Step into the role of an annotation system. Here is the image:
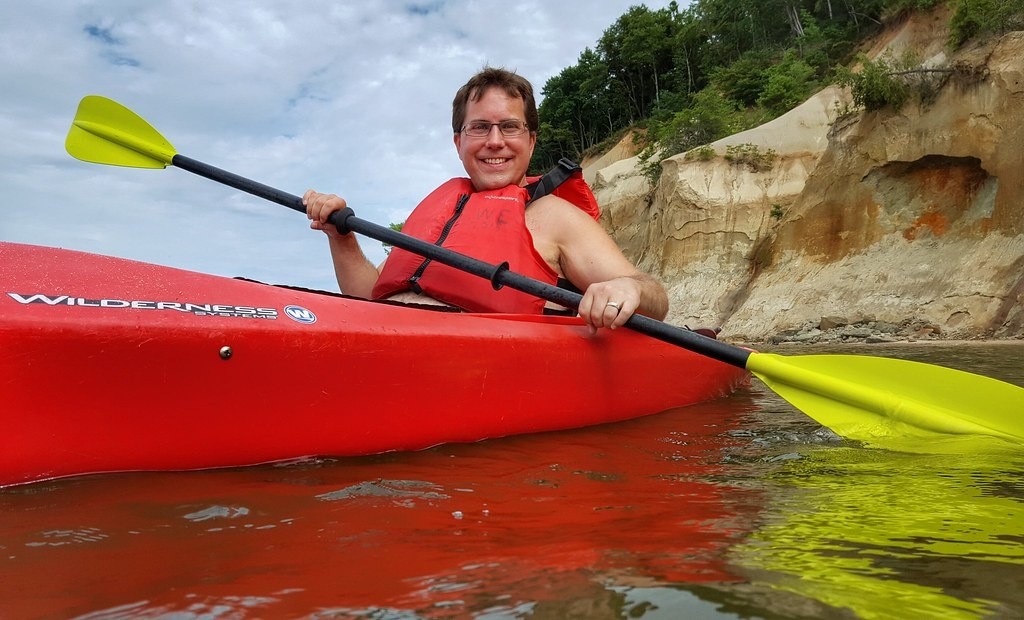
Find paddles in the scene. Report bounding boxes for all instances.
[63,95,1023,441]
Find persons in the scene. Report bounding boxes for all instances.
[302,58,669,335]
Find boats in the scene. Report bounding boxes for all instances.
[0,241,759,489]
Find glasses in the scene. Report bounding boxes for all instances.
[458,119,530,137]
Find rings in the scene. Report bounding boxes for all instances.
[606,301,620,310]
